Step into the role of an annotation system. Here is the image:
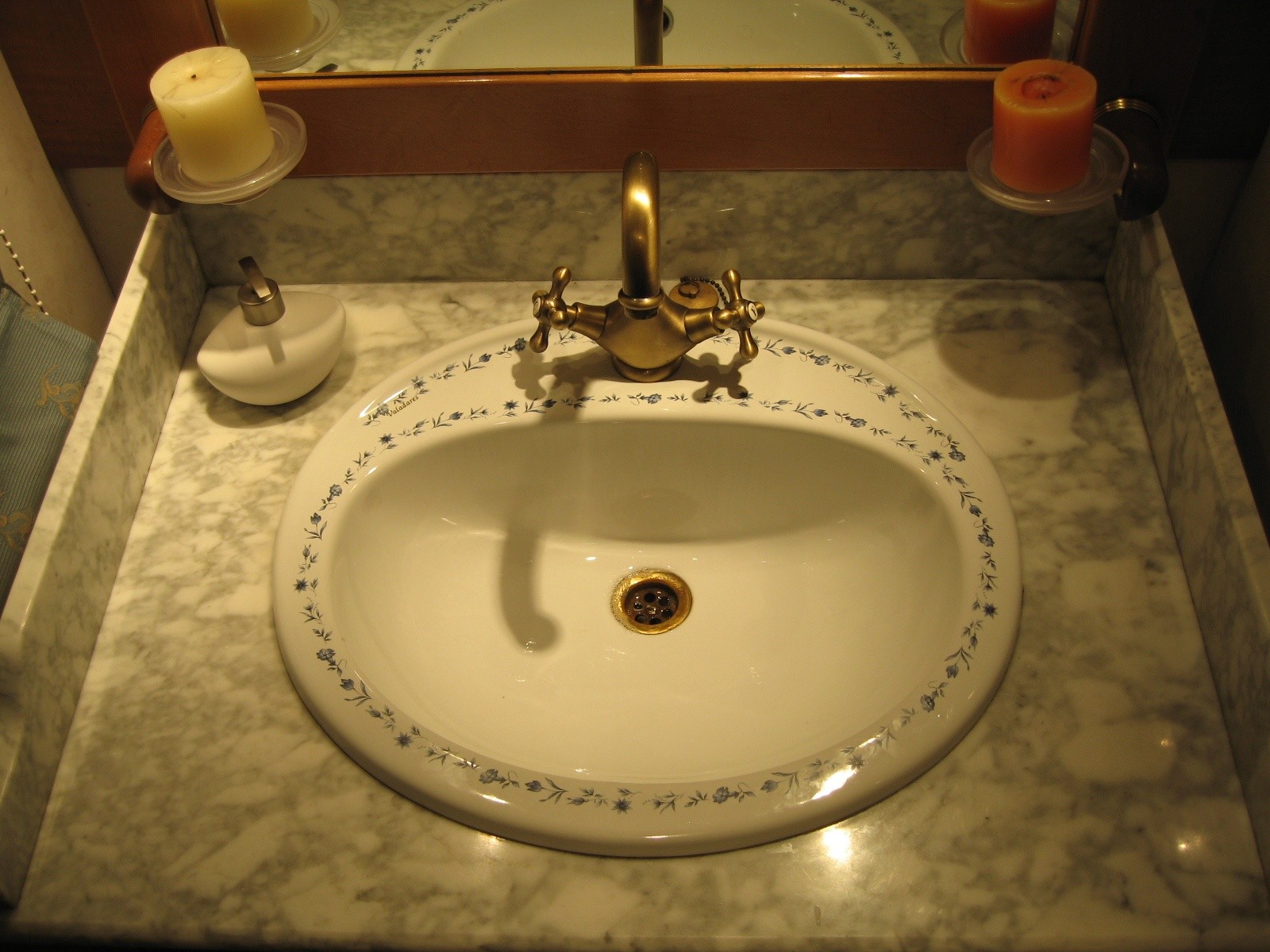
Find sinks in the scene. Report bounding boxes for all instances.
[393,0,924,71]
[267,400,1028,860]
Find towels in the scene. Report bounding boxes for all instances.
[0,283,100,616]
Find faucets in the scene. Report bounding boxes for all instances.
[528,148,766,385]
[633,0,663,66]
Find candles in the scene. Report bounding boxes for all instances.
[963,0,1054,65]
[215,1,313,59]
[151,47,275,183]
[991,58,1097,194]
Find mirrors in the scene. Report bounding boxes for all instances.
[81,0,1214,177]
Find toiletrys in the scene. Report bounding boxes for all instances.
[196,255,346,406]
